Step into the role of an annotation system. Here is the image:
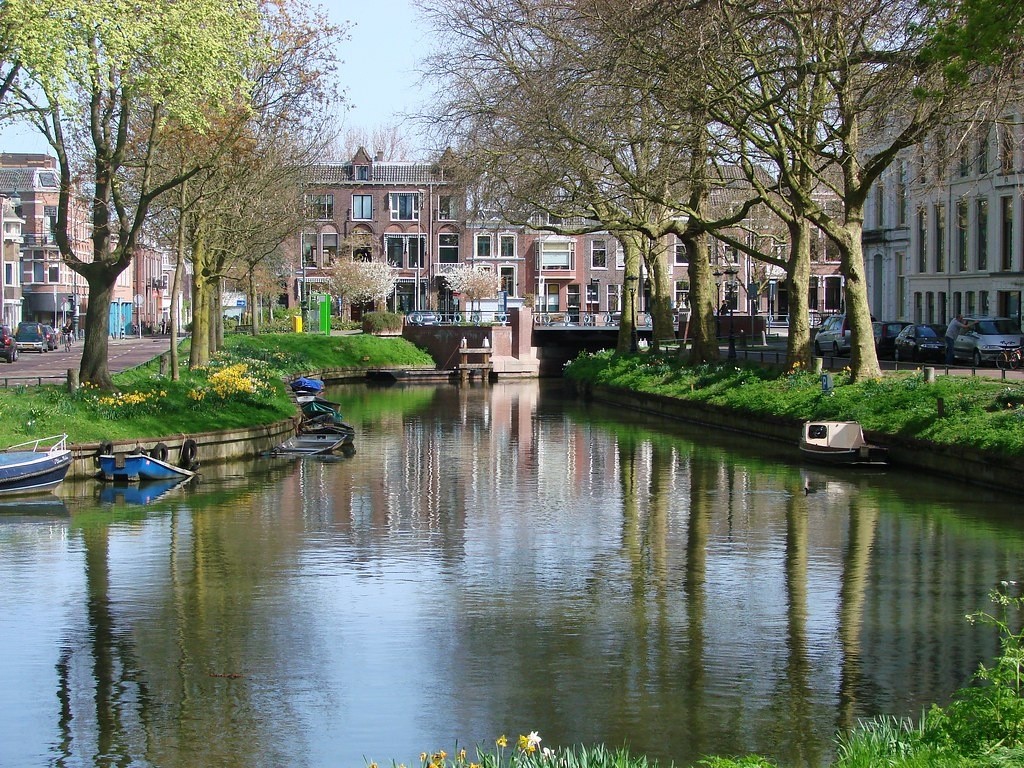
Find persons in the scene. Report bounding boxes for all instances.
[718,300,729,317]
[945,313,979,364]
[62,318,72,345]
[161,319,166,335]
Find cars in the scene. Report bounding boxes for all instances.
[872,321,914,360]
[894,324,948,363]
[44,325,58,351]
[407,311,437,325]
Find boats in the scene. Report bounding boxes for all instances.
[290,377,325,392]
[259,433,347,458]
[799,420,890,471]
[99,452,195,482]
[302,400,341,419]
[0,434,72,497]
[295,391,327,405]
[300,412,355,445]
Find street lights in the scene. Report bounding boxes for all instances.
[712,269,724,341]
[0,187,21,327]
[626,274,639,352]
[723,268,739,359]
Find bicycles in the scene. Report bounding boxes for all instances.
[996,340,1022,370]
[63,333,71,353]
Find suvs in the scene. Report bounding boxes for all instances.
[0,324,19,363]
[15,322,48,353]
[946,313,1024,367]
[814,314,878,358]
